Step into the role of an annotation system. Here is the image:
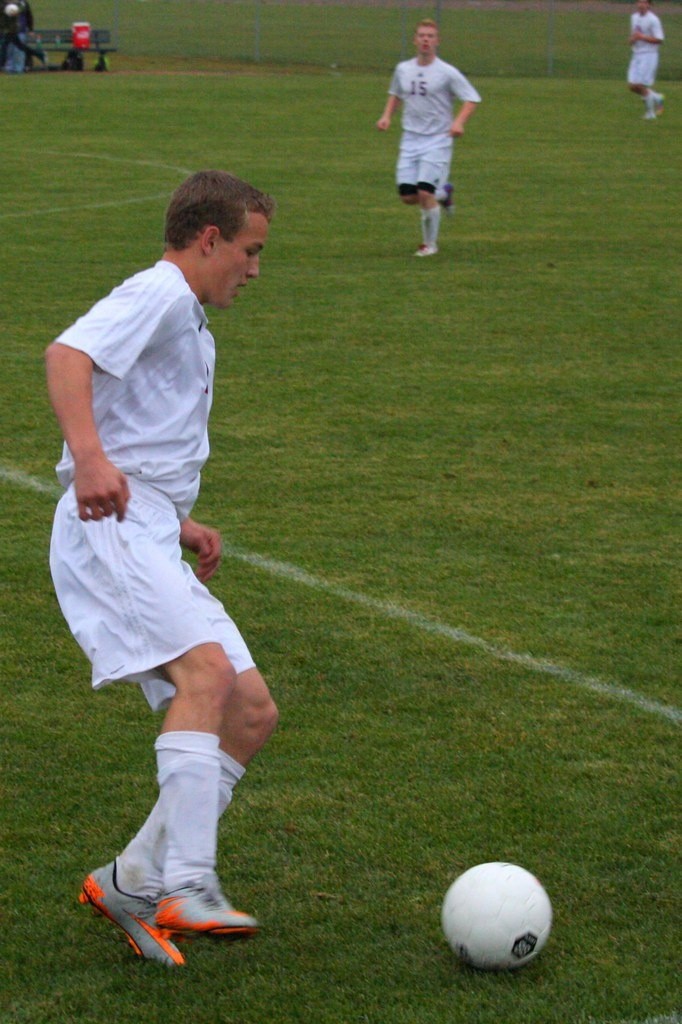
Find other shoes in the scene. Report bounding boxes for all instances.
[415,245,437,258]
[657,95,666,115]
[644,114,655,120]
[440,186,455,217]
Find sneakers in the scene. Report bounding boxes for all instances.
[155,873,261,938]
[79,856,185,969]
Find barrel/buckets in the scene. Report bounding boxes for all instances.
[71,21,92,48]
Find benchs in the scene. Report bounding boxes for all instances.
[27,30,118,72]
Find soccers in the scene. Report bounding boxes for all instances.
[439,860,555,971]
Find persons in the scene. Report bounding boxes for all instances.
[625,0,665,121]
[46,168,280,966]
[0,0,47,72]
[375,18,482,258]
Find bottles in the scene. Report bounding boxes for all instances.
[55,35,60,47]
[36,35,42,48]
